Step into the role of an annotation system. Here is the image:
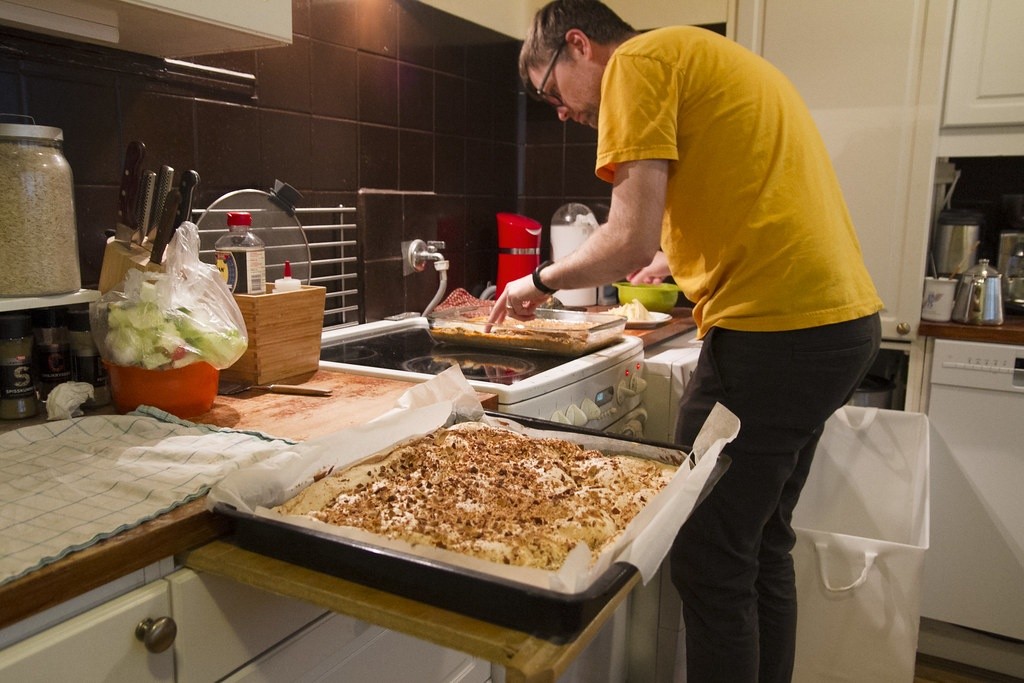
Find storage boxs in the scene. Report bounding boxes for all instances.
[224,281,327,387]
[787,405,930,681]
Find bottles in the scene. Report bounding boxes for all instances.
[272,261,306,296]
[213,212,267,298]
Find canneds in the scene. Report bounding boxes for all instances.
[0,123,81,296]
[0,307,113,422]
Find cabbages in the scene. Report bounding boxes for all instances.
[101,280,250,369]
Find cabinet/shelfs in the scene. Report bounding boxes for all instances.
[734,0,954,344]
[0,0,292,59]
[624,327,703,682]
[0,579,175,683]
[161,566,492,683]
[935,0,1023,157]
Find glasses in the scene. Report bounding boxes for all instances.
[536,38,565,107]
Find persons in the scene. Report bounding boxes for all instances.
[486,0,885,683]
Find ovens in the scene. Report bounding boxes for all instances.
[494,337,647,683]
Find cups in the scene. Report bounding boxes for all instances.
[920,276,960,321]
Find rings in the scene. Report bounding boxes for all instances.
[505,303,513,309]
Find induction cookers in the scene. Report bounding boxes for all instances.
[314,311,644,391]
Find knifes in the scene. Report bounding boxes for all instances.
[116,139,147,250]
[169,169,203,241]
[130,170,156,246]
[149,189,180,264]
[151,163,176,238]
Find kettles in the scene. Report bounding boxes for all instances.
[492,210,544,306]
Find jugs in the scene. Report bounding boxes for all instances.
[952,258,1005,326]
[933,211,982,277]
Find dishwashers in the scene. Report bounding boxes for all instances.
[915,328,1024,683]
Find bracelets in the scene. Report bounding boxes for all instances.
[531,258,560,294]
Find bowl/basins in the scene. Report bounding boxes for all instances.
[99,359,221,418]
[848,376,896,411]
[611,281,685,313]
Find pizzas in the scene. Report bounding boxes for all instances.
[279,422,682,575]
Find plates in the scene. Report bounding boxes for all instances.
[595,310,673,329]
